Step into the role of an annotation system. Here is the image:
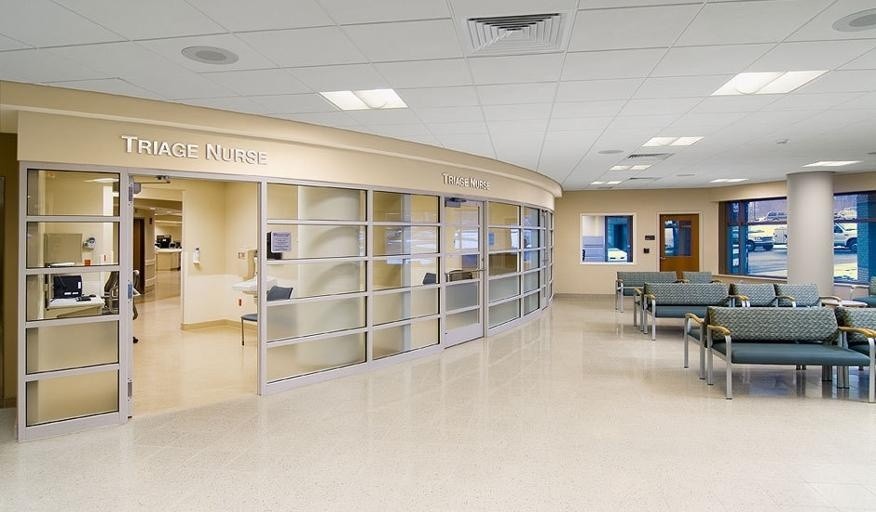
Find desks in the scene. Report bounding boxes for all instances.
[43,294,103,320]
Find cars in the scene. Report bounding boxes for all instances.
[607,249,628,262]
[664,243,674,256]
[733,208,858,254]
[386,226,528,269]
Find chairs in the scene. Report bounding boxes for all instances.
[101,270,140,345]
[240,286,292,346]
[421,269,473,286]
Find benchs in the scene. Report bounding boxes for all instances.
[614,268,876,403]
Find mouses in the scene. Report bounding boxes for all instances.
[90,293,96,297]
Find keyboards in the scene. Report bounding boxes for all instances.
[77,295,91,301]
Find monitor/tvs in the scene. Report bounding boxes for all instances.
[54,275,82,298]
